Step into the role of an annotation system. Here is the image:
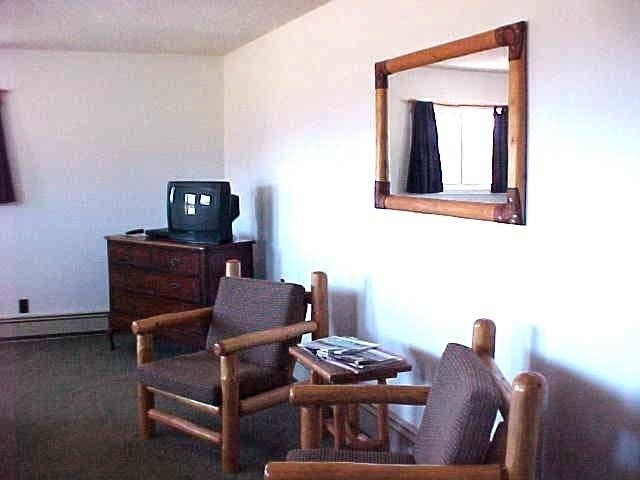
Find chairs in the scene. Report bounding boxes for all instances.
[264,318,546,479]
[131,258,328,474]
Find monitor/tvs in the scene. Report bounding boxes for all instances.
[167,181,239,243]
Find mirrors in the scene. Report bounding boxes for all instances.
[374,21,527,227]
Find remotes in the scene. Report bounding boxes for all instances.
[127,229,145,233]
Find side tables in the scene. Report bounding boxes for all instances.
[288,334,412,451]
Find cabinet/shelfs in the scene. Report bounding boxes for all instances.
[103,231,256,350]
